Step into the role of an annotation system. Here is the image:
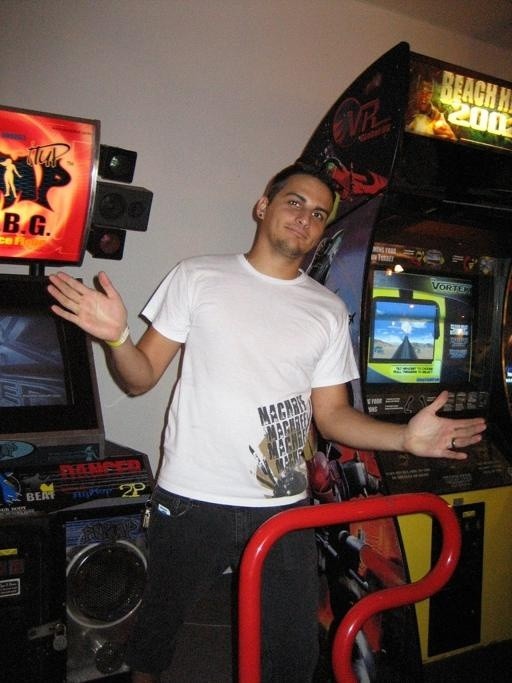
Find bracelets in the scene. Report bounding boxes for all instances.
[105,327,130,347]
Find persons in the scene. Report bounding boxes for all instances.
[47,163,488,683]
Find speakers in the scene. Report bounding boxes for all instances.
[86,227,126,261]
[98,143,138,184]
[91,181,153,231]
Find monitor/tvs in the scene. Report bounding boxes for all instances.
[365,270,486,391]
[1,298,98,434]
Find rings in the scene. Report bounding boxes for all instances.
[452,437,456,448]
